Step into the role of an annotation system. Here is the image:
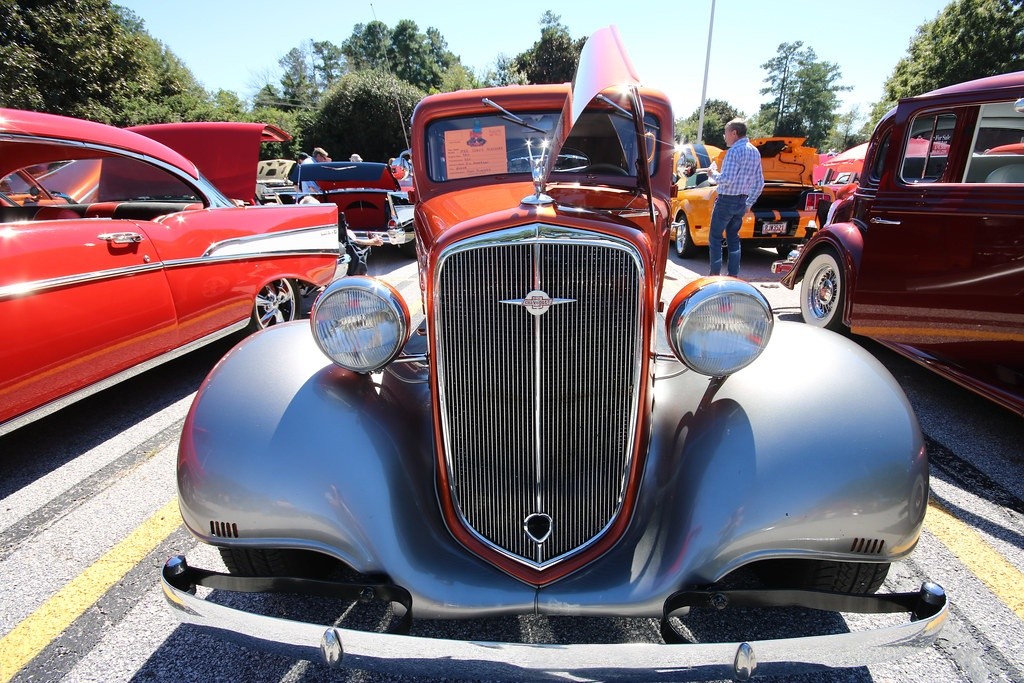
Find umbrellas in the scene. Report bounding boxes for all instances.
[821,137,951,174]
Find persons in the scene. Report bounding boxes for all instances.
[350,154,362,163]
[708,121,764,278]
[389,151,412,166]
[288,147,332,186]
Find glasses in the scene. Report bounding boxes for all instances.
[321,155,326,160]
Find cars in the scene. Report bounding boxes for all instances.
[0,104,352,437]
[665,136,834,259]
[155,30,951,670]
[287,158,420,258]
[770,66,1024,420]
